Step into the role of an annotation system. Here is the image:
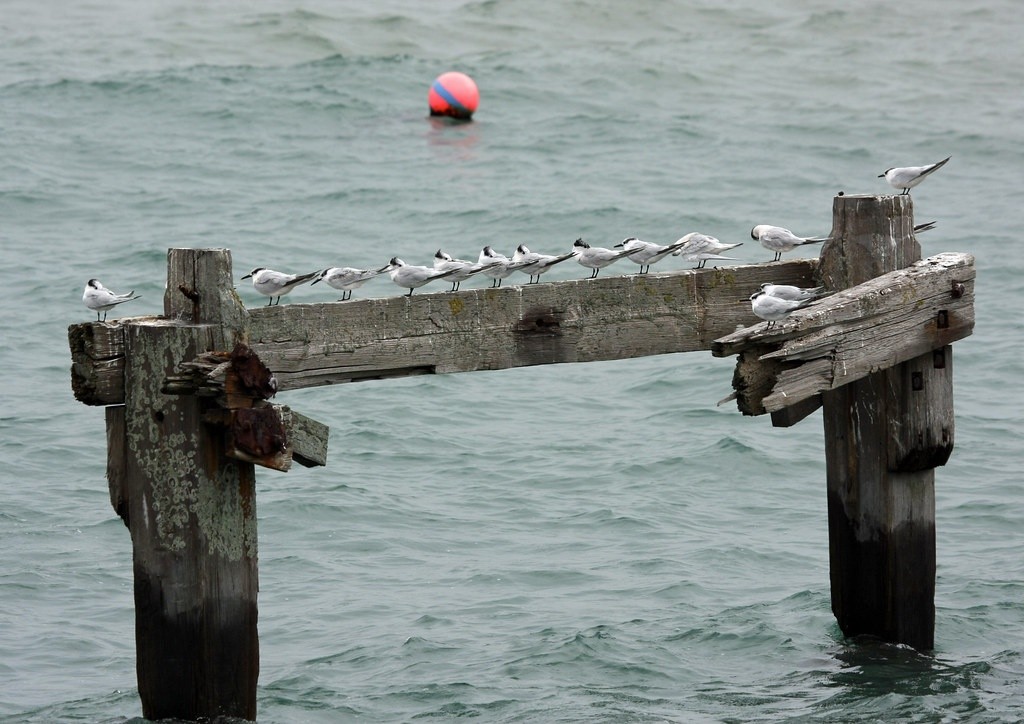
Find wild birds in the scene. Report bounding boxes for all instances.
[510,244,580,284]
[750,224,835,263]
[378,256,465,299]
[81,275,141,326]
[613,236,691,276]
[476,244,540,288]
[739,282,825,331]
[239,264,330,306]
[672,231,745,269]
[571,237,647,278]
[914,219,938,235]
[877,155,953,195]
[433,247,502,292]
[309,263,401,302]
